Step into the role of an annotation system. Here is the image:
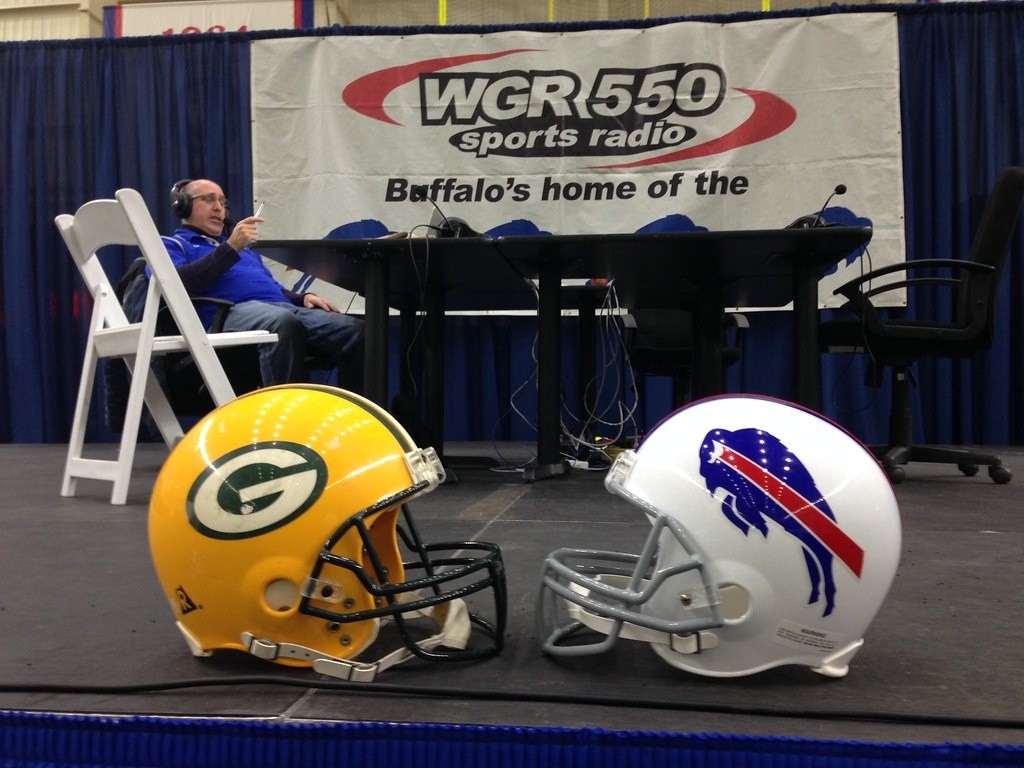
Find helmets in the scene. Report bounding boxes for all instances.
[536,393,902,677]
[148,383,508,682]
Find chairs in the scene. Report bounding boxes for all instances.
[55,188,280,506]
[129,252,334,414]
[818,166,1024,483]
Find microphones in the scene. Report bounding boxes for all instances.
[811,184,847,227]
[414,186,454,231]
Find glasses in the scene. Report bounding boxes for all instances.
[192,194,230,206]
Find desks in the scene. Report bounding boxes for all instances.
[248,225,872,482]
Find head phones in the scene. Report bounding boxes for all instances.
[438,212,490,238]
[170,179,193,221]
[784,214,825,231]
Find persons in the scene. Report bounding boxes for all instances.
[145,177,366,395]
